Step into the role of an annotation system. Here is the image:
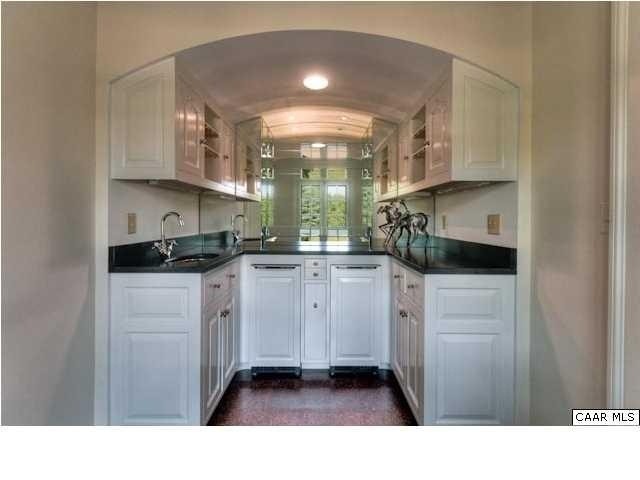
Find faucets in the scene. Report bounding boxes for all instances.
[152,211,184,260]
[230,214,249,248]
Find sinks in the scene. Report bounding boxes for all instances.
[242,237,261,242]
[164,252,220,264]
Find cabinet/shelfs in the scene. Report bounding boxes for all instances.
[329,256,387,374]
[243,254,301,375]
[301,255,328,370]
[111,258,241,425]
[109,57,261,202]
[374,56,520,201]
[389,257,515,425]
